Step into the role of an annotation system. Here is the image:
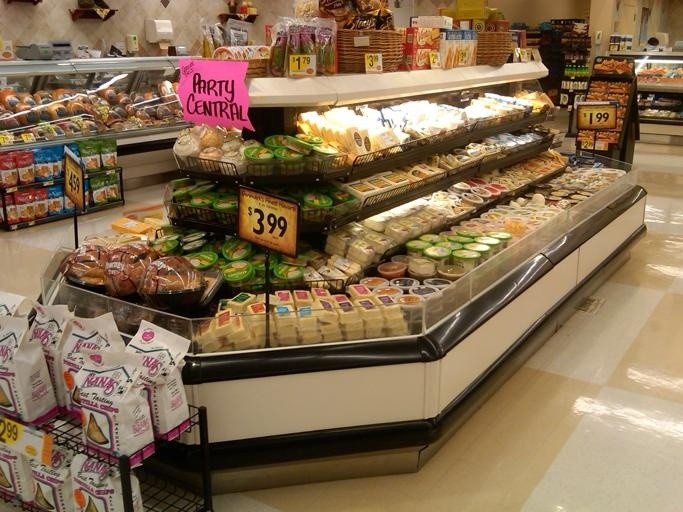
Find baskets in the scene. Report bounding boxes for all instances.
[478,30,512,68]
[337,28,405,72]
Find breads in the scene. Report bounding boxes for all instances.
[60,239,207,296]
